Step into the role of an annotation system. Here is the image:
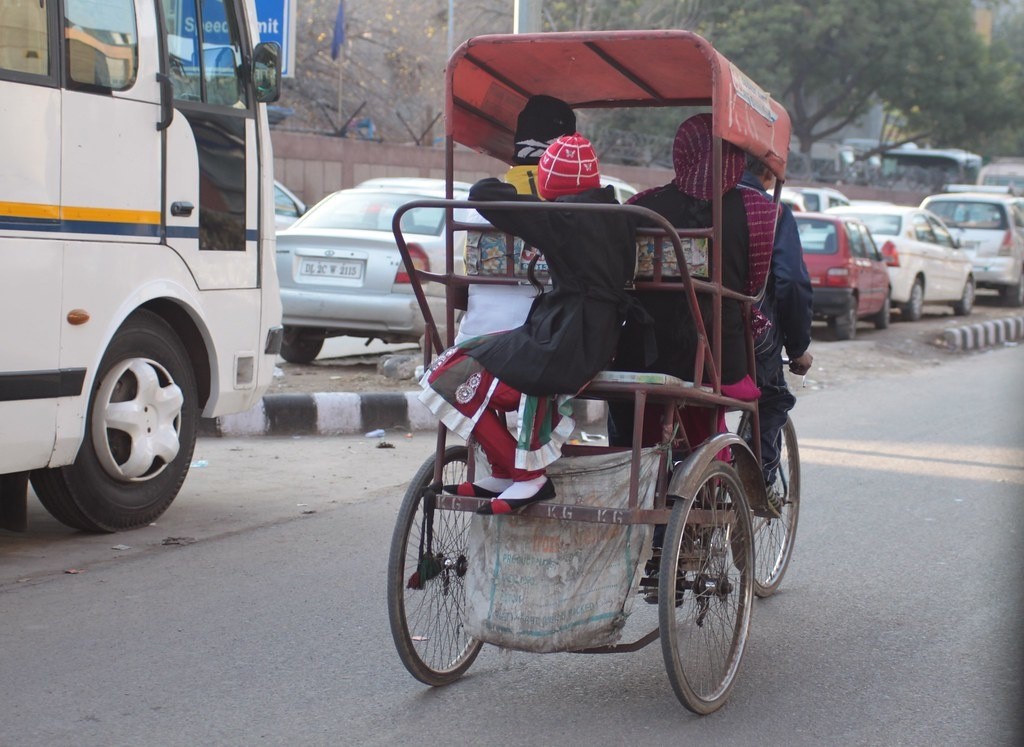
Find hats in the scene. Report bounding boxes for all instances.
[512,95,577,163]
[538,133,601,200]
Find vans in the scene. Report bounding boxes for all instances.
[919,192,1024,306]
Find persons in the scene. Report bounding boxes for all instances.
[415,94,638,516]
[608,113,817,608]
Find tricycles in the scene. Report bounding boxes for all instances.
[388,29,800,715]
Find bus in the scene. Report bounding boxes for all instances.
[786,137,982,194]
[0,0,284,534]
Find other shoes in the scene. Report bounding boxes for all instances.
[753,485,781,518]
[477,478,555,515]
[444,483,504,497]
[644,569,684,607]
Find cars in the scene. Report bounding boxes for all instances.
[274,174,638,363]
[791,211,892,339]
[766,186,852,212]
[976,162,1024,197]
[824,205,976,321]
